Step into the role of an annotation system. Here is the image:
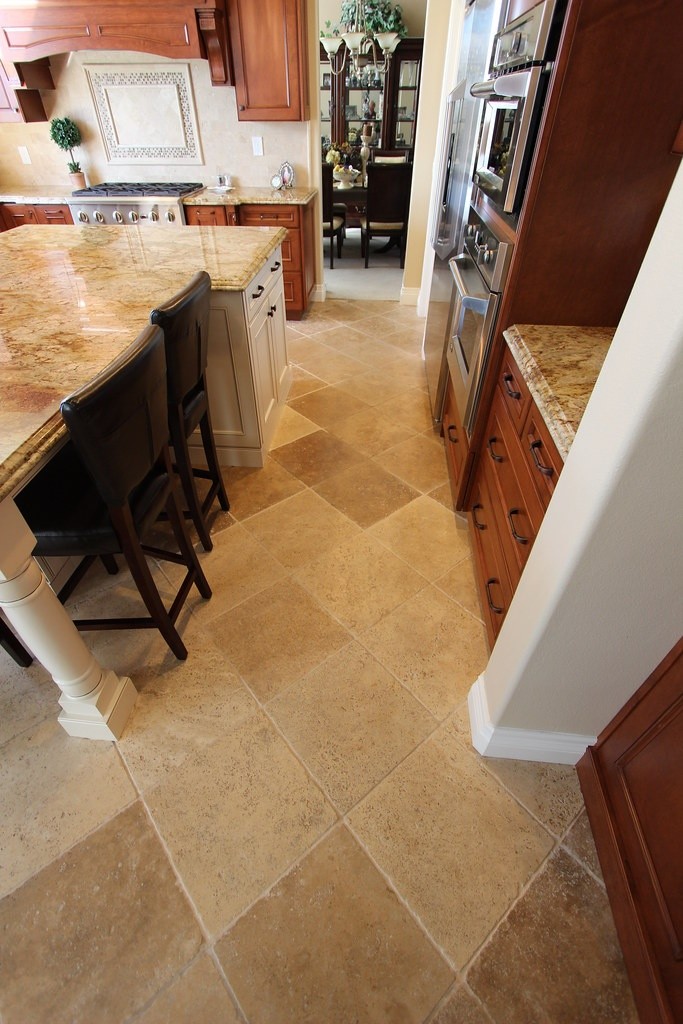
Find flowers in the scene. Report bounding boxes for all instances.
[333,161,358,173]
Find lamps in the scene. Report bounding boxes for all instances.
[319,0,402,75]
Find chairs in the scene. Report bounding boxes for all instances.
[0,319,216,669]
[358,161,413,269]
[156,265,236,552]
[323,162,346,270]
[370,148,411,163]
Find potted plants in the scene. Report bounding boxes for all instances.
[47,115,86,189]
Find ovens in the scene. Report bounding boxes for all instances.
[445,182,524,450]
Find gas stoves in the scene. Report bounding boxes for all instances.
[66,182,204,228]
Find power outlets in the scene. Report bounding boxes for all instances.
[18,146,32,165]
[251,136,265,156]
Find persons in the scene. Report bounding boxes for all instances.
[282,167,290,184]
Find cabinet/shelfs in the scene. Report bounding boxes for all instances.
[440,370,474,515]
[0,203,73,230]
[173,243,287,471]
[238,204,316,322]
[459,339,566,653]
[188,207,238,226]
[0,0,235,124]
[224,0,314,122]
[320,36,425,160]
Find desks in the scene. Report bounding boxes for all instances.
[332,187,368,228]
[0,221,293,744]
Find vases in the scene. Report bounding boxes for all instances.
[334,172,361,190]
[358,136,371,172]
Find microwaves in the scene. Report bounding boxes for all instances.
[470,1,574,214]
